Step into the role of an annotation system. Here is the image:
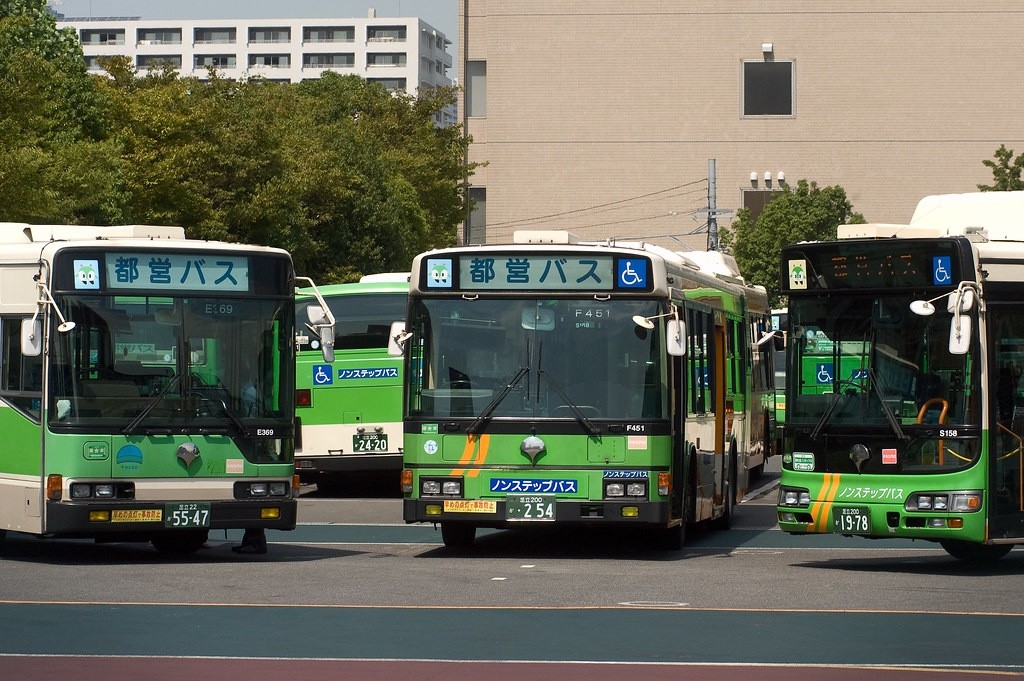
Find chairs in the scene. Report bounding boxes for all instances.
[109,361,174,383]
[82,383,140,397]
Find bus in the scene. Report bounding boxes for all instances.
[774,188,1024,566]
[761,307,872,433]
[0,220,335,561]
[386,230,777,552]
[271,271,413,498]
[76,293,226,394]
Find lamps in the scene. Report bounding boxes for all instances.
[764,172,772,181]
[751,172,758,180]
[777,171,785,181]
